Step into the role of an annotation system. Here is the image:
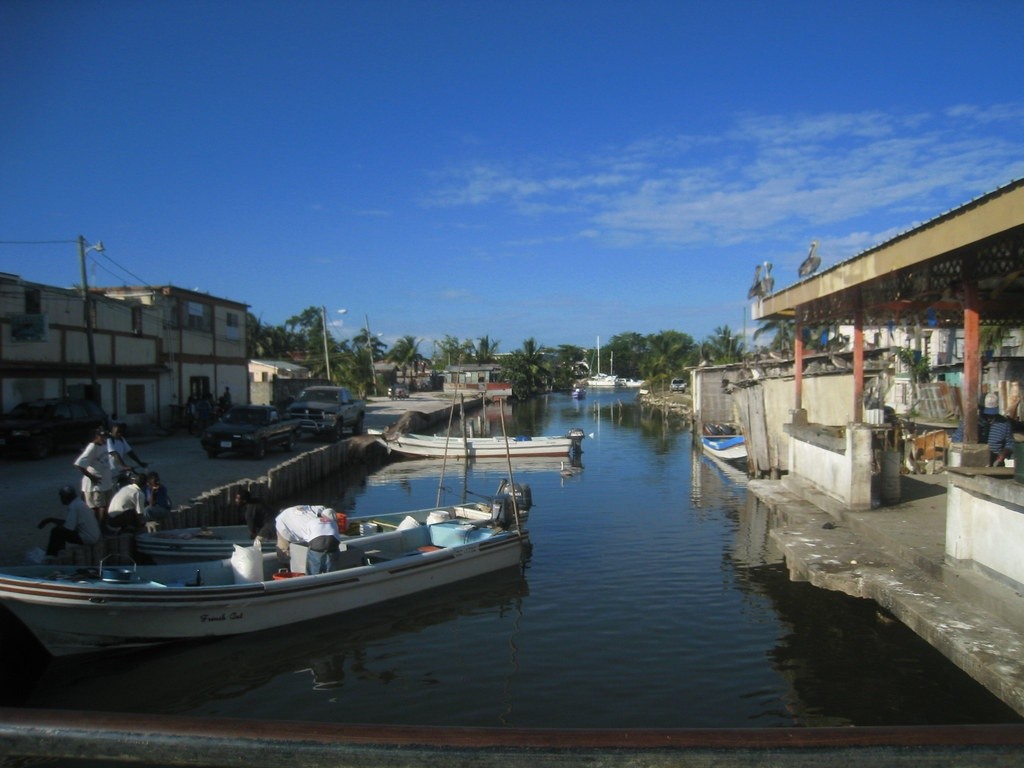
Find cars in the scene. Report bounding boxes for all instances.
[1,399,109,460]
[669,378,687,393]
[387,383,410,398]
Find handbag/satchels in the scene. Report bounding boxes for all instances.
[22,547,47,565]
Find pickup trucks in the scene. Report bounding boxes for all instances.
[199,405,304,461]
[286,384,365,444]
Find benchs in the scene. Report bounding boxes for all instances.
[374,518,401,529]
[351,547,407,561]
[430,523,495,547]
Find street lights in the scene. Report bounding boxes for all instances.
[321,304,348,381]
[364,313,382,396]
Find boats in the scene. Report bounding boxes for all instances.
[133,354,533,566]
[1,399,531,688]
[367,428,584,462]
[701,435,747,461]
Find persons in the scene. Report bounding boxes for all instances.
[107,473,146,532]
[106,423,149,489]
[73,426,116,531]
[951,392,1016,468]
[185,386,230,435]
[36,485,102,563]
[145,471,171,521]
[275,503,340,575]
[232,487,276,544]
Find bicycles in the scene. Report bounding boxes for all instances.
[120,465,173,515]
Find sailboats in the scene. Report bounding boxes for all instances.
[587,335,619,386]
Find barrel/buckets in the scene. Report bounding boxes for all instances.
[99,553,136,582]
[430,510,449,524]
[396,515,420,531]
[272,568,306,580]
[359,522,378,537]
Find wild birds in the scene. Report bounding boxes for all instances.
[748,261,775,300]
[798,240,821,278]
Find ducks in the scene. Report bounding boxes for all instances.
[560,461,583,475]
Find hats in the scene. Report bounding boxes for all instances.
[95,425,106,435]
[58,486,75,496]
[978,392,1000,415]
